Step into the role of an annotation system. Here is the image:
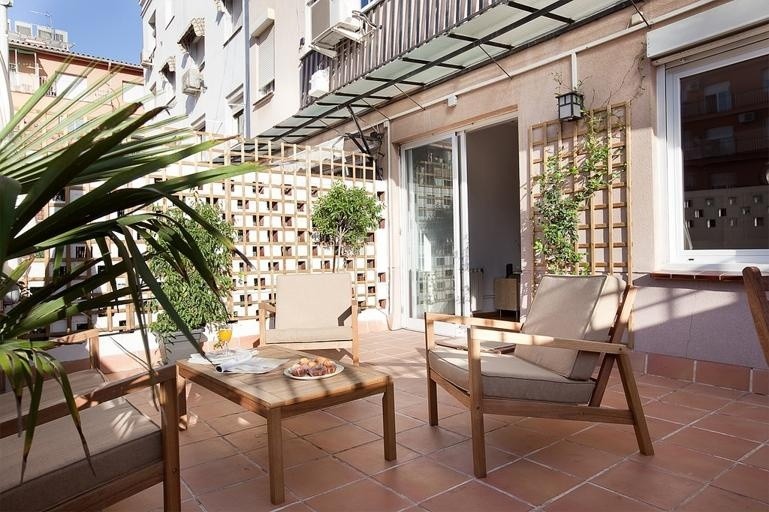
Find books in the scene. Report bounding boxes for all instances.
[215,353,289,375]
[188,349,257,365]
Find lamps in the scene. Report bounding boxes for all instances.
[557,91,584,123]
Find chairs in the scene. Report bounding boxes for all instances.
[1,329,181,511]
[742,267,767,361]
[424,275,654,480]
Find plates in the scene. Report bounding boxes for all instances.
[283,363,344,380]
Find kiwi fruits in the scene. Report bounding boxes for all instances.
[291,356,336,377]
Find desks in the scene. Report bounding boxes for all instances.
[175,344,398,507]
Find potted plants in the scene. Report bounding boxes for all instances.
[145,195,237,366]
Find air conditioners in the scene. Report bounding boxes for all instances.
[182,68,203,95]
[307,0,376,59]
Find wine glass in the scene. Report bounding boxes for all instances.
[218,324,233,360]
